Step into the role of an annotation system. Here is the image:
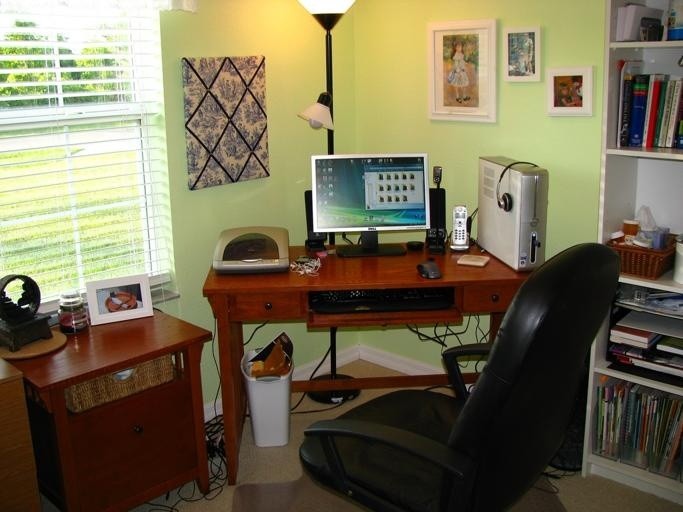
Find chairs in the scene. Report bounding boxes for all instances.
[298,243,622,512]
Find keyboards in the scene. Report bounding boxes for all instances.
[309,286,453,314]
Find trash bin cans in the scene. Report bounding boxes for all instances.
[241,348,294,447]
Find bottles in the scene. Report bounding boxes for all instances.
[622,219,638,245]
[609,229,623,246]
[57,298,89,335]
[631,239,648,249]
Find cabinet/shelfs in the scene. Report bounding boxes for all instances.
[23,308,212,512]
[581,0,683,506]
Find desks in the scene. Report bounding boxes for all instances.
[202,240,533,487]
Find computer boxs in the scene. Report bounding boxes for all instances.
[477,155,549,272]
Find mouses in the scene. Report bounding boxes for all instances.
[418,262,442,279]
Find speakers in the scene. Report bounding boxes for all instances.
[304,190,327,251]
[426,186,446,255]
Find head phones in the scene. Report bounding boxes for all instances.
[495,161,539,211]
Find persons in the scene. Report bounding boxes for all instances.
[616,0,683,41]
[607,310,683,377]
[595,378,683,481]
[445,40,472,102]
[618,60,683,149]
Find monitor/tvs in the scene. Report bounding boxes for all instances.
[311,154,431,257]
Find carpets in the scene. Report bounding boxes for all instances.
[231,473,565,512]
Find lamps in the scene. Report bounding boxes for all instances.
[298,0,356,245]
[297,91,335,130]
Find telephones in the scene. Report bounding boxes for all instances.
[450,205,469,250]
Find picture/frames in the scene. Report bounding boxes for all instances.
[428,18,497,123]
[544,65,593,117]
[181,55,270,191]
[85,274,154,326]
[503,26,541,83]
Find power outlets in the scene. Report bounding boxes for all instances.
[208,452,227,476]
